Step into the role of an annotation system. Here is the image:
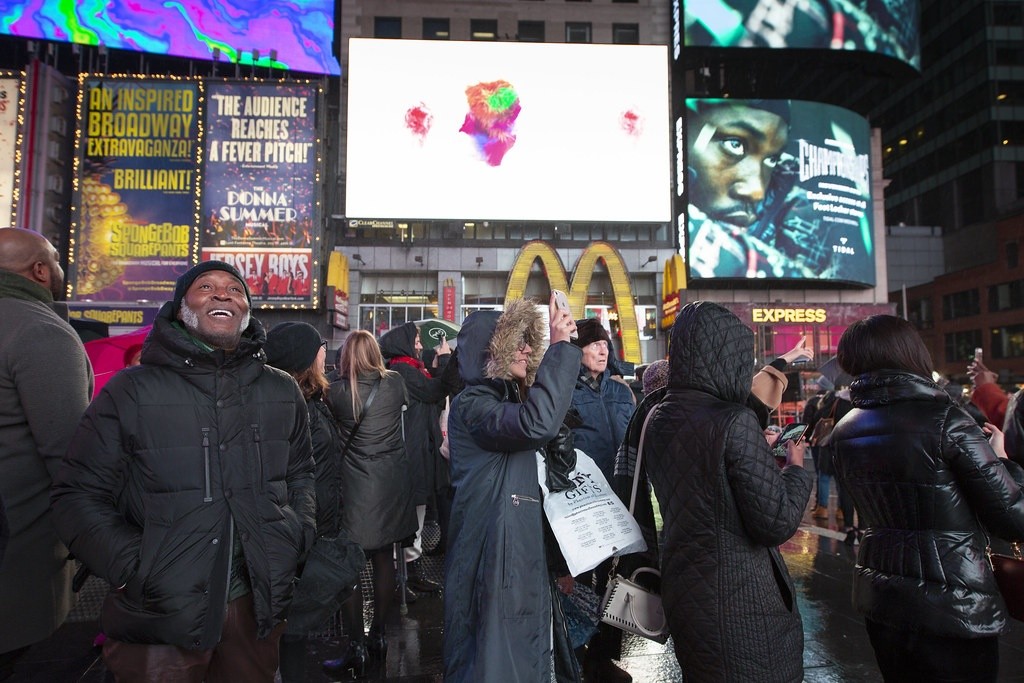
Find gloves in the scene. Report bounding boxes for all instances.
[548,424,578,491]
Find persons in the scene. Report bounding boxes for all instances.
[1,225,98,682]
[436,290,584,681]
[556,317,642,683]
[49,260,322,682]
[691,97,836,280]
[641,301,815,682]
[828,313,1023,682]
[72,303,1022,607]
[323,329,422,671]
[264,320,367,681]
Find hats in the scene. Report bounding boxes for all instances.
[176,261,252,321]
[263,320,321,372]
[641,359,671,396]
[571,319,611,349]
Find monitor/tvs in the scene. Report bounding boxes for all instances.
[0,1,340,77]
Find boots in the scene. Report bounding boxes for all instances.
[405,557,443,590]
[428,518,450,559]
[391,571,417,603]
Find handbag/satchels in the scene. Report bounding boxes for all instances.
[983,551,1024,621]
[807,416,837,447]
[534,447,649,578]
[597,564,672,648]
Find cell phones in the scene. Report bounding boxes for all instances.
[973,347,983,364]
[550,288,578,342]
[437,332,443,347]
[772,422,810,457]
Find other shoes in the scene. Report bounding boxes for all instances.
[581,650,633,682]
[811,503,864,545]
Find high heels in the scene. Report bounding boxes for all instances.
[324,642,374,679]
[366,630,385,661]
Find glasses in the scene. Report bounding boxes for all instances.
[321,340,330,350]
[516,335,532,352]
